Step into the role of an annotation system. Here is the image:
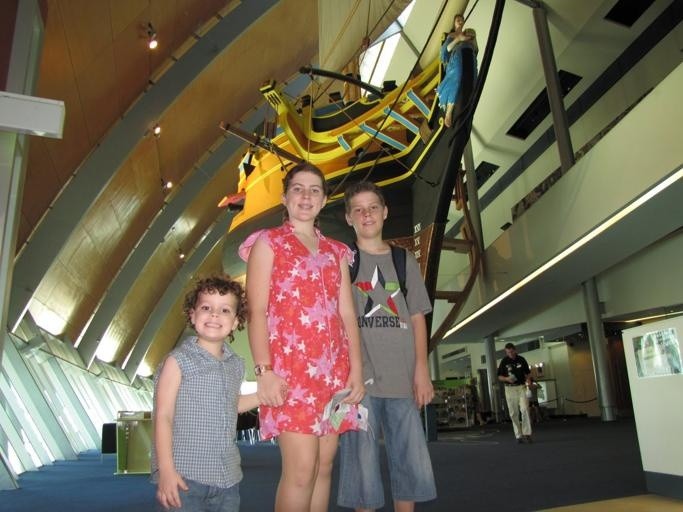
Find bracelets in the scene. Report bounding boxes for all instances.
[250,364,274,374]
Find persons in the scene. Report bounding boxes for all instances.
[521,376,543,424]
[246,159,367,511]
[340,73,356,108]
[338,182,440,511]
[151,277,281,511]
[435,13,479,128]
[496,342,535,443]
